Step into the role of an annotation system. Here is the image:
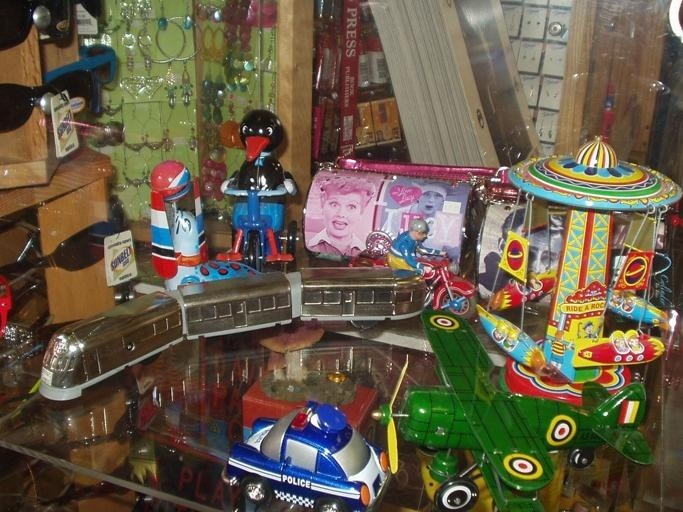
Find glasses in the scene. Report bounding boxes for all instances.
[1,68,93,134]
[0,194,129,275]
[41,43,118,88]
[0,0,75,54]
